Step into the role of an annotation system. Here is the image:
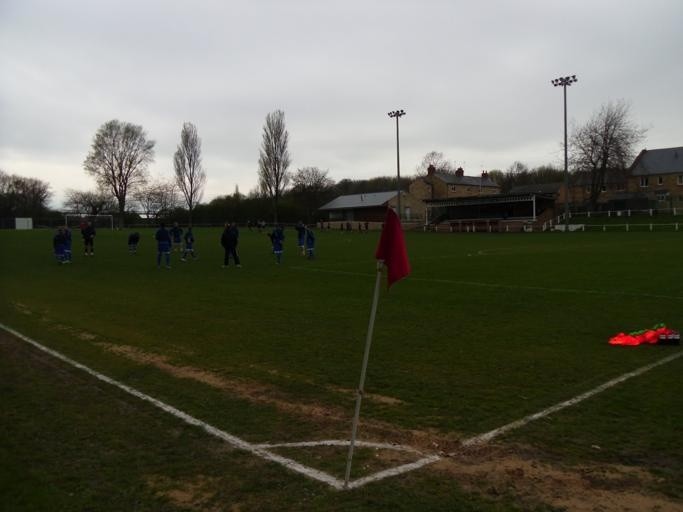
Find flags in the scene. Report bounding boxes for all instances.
[375,208,411,293]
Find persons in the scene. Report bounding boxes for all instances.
[153,218,317,269]
[128,231,141,254]
[52,226,65,263]
[60,225,73,265]
[81,220,95,257]
